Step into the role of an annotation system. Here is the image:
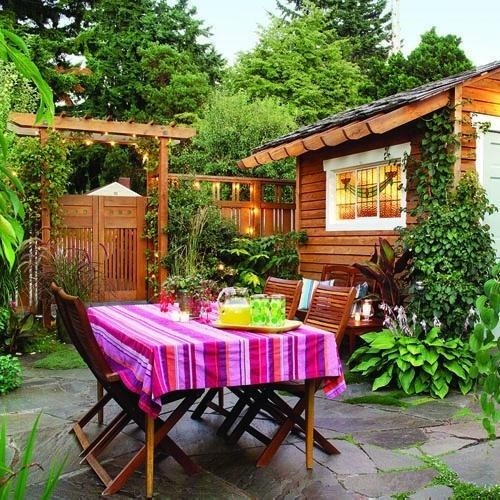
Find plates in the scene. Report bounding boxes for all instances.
[212,319,303,333]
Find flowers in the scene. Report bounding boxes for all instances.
[160,277,213,323]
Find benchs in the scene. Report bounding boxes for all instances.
[295,263,380,320]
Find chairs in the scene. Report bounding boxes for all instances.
[51,282,205,497]
[225,284,357,470]
[190,277,303,436]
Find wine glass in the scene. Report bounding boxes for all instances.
[360,303,375,322]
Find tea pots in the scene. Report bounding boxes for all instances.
[217,286,250,325]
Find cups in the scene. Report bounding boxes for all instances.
[250,294,287,328]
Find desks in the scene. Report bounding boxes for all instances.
[345,316,385,354]
